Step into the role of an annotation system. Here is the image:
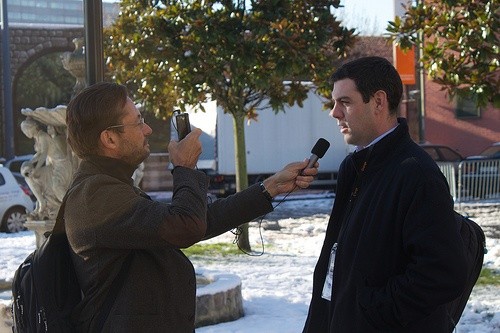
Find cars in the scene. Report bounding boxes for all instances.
[0,153,37,234]
[419,142,500,196]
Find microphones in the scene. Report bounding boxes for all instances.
[302,138,330,175]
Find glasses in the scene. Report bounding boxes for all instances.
[105,118,144,131]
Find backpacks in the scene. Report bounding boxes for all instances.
[377,156,487,333]
[11,174,149,333]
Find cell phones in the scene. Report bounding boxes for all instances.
[176,113,191,141]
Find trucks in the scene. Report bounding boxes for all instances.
[167,80,356,197]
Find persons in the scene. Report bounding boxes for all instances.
[20,119,72,217]
[302,56,455,333]
[63,81,320,333]
